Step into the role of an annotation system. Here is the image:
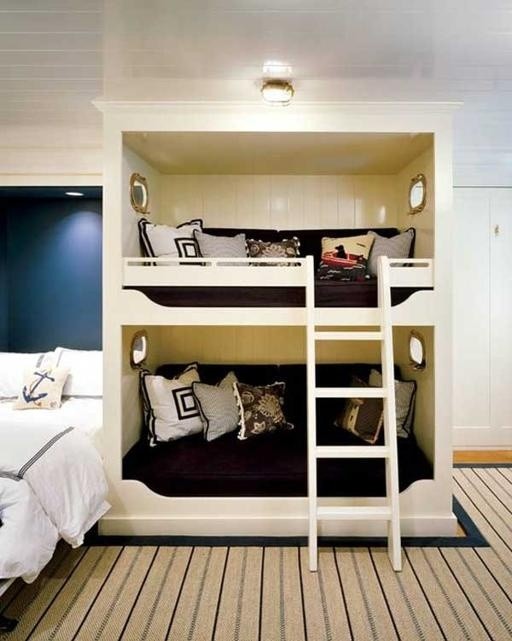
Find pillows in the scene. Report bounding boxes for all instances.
[317,227,419,275]
[0,347,105,413]
[331,367,420,445]
[135,214,302,270]
[133,364,295,442]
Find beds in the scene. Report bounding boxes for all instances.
[0,339,105,621]
[110,225,434,328]
[119,362,436,502]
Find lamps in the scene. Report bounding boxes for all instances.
[406,173,427,214]
[128,329,148,371]
[128,172,152,215]
[405,330,428,374]
[260,81,298,107]
